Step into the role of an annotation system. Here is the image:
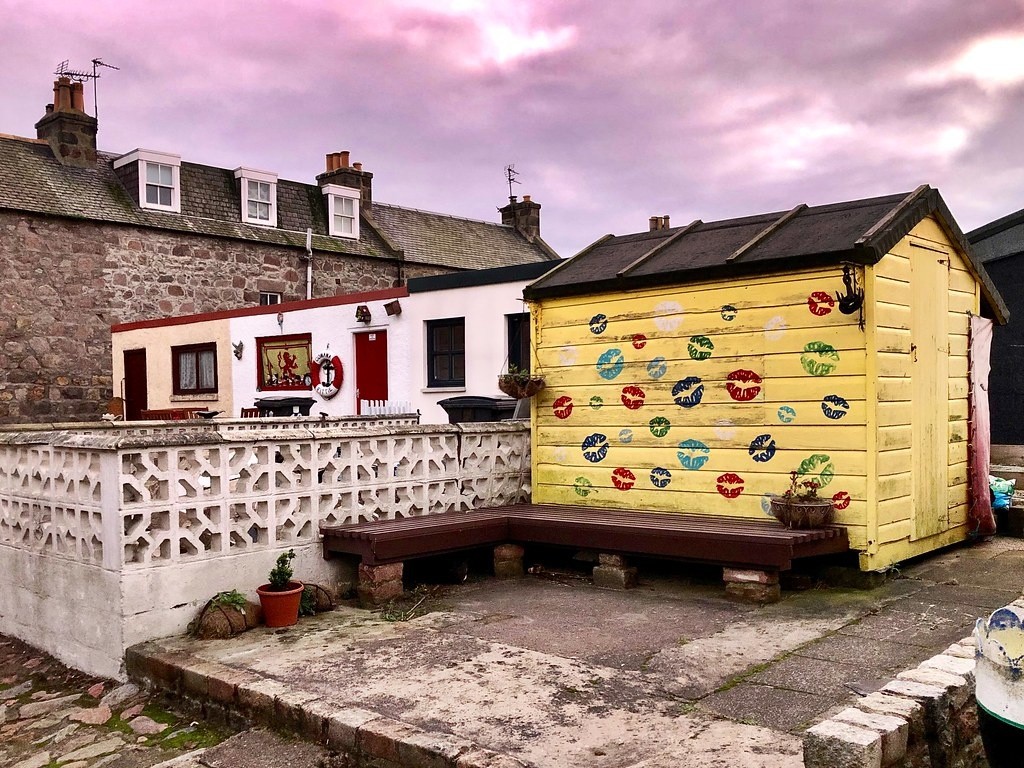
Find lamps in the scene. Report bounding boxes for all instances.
[383,300,400,316]
[356,304,371,325]
[277,311,284,326]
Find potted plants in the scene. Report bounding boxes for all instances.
[497,364,545,401]
[255,550,302,627]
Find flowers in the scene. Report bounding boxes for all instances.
[780,472,837,503]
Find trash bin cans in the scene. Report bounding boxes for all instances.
[437,395,518,425]
[253,396,318,418]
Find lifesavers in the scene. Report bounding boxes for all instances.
[309,349,344,398]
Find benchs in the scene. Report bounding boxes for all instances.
[321,503,851,600]
[228,408,267,430]
[141,407,211,421]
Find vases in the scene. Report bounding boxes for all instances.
[769,500,834,530]
